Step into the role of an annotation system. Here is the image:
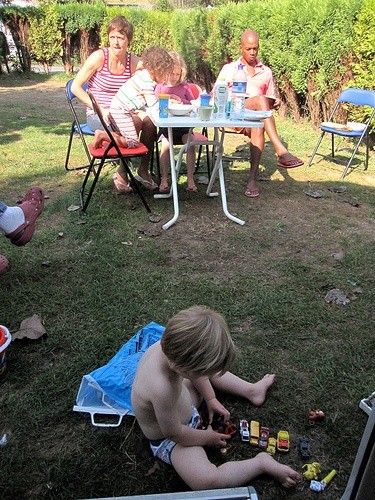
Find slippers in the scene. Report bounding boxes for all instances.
[277,157,304,168]
[134,168,158,191]
[112,172,133,193]
[245,184,260,197]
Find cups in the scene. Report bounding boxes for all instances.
[199,107,212,121]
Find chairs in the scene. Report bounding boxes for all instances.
[305,88,375,181]
[63,78,253,213]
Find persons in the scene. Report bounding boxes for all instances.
[158,51,198,192]
[131,305,301,491]
[70,16,157,195]
[0,186,45,276]
[93,46,173,149]
[210,30,304,197]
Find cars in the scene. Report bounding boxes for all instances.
[276,430,290,453]
[266,437,277,456]
[259,427,270,448]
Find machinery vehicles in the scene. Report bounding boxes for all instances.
[240,419,250,442]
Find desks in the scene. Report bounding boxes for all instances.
[142,100,264,231]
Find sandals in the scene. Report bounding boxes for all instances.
[0,255,9,275]
[4,186,44,246]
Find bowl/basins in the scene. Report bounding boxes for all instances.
[168,104,193,115]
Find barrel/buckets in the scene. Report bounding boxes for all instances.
[0,325,12,374]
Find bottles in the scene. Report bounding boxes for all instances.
[158,93,170,118]
[217,77,228,120]
[231,63,247,121]
[200,94,211,106]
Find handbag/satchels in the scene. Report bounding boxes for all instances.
[72,321,166,427]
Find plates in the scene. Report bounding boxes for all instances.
[245,111,272,120]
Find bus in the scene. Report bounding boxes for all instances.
[249,420,259,447]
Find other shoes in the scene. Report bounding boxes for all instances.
[187,176,198,193]
[159,177,171,193]
